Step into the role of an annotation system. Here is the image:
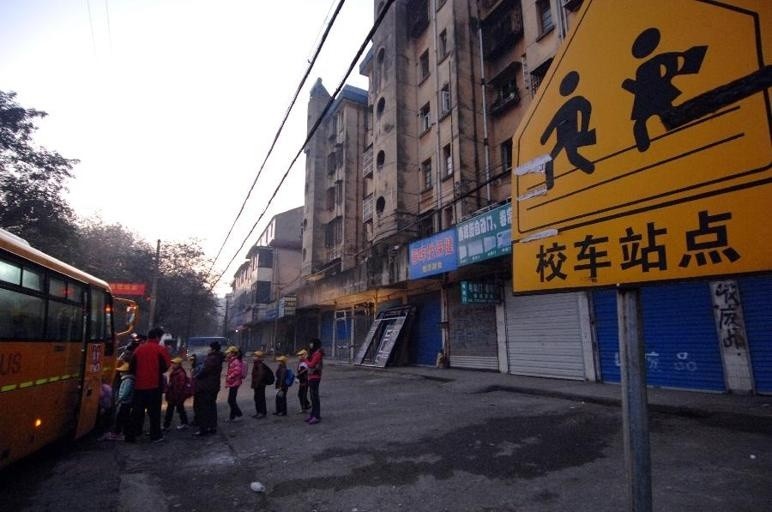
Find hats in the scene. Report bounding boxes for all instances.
[254,351,263,357]
[225,346,238,354]
[295,349,307,356]
[115,362,129,371]
[276,356,288,362]
[171,357,183,365]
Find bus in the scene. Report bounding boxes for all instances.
[0,225,140,480]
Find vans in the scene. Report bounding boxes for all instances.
[188,335,236,358]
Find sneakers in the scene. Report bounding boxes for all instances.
[304,414,319,423]
[272,412,287,416]
[176,424,189,430]
[223,416,244,422]
[252,412,266,419]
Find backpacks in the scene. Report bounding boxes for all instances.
[241,360,248,379]
[183,375,192,399]
[285,369,295,386]
[262,364,275,385]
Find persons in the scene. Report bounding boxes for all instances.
[296,349,311,413]
[273,355,289,416]
[106,362,136,441]
[192,341,225,436]
[119,334,146,359]
[303,337,323,424]
[189,352,205,426]
[168,342,192,357]
[120,327,171,444]
[250,350,267,418]
[160,357,190,431]
[224,345,244,423]
